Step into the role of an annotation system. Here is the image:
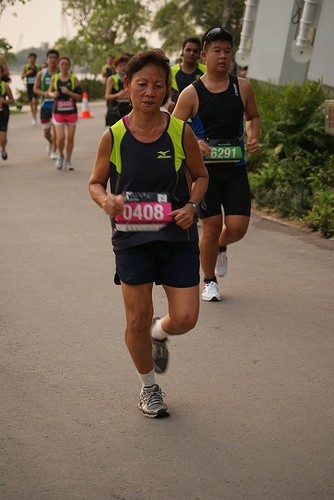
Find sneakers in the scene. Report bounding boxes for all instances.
[201,281,221,302]
[151,317,170,373]
[214,252,228,277]
[138,384,170,418]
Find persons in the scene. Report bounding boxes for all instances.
[0,65,14,160]
[88,47,209,418]
[172,25,261,301]
[33,49,60,160]
[48,57,82,170]
[238,66,249,78]
[41,62,48,69]
[21,53,40,124]
[105,54,134,128]
[101,53,114,81]
[165,37,209,114]
[174,58,181,64]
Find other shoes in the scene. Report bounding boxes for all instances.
[56,157,64,169]
[65,160,74,171]
[47,143,52,154]
[51,152,57,160]
[1,150,7,160]
[32,119,37,126]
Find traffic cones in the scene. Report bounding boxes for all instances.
[77,92,95,119]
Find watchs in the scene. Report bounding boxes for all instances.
[187,201,199,212]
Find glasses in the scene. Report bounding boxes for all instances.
[203,28,232,52]
